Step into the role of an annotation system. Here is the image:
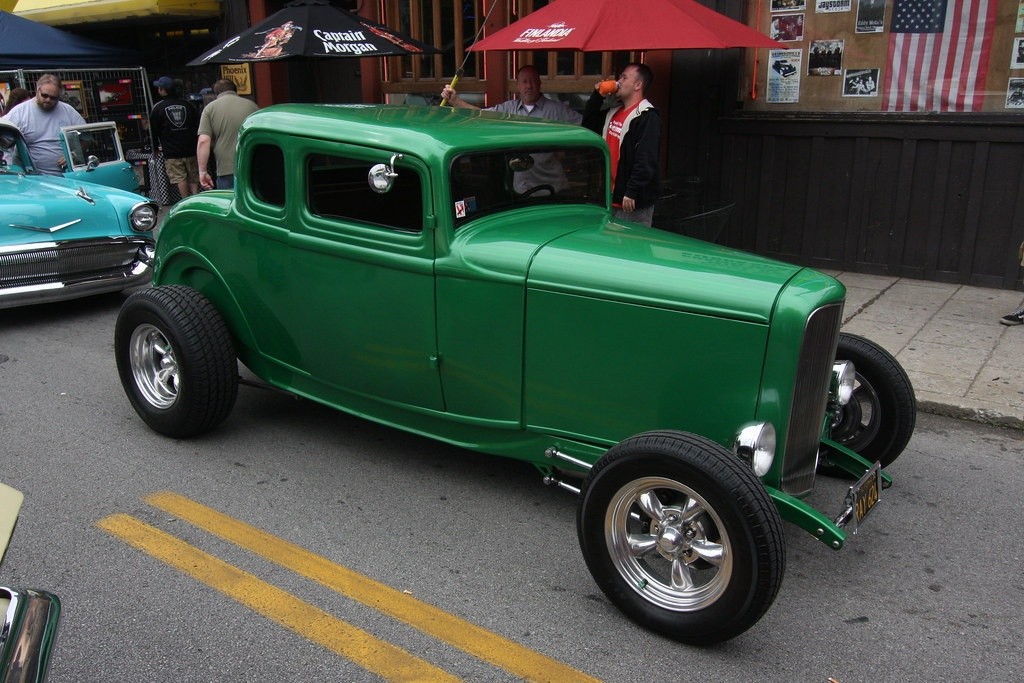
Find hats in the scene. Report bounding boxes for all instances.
[153,76,173,89]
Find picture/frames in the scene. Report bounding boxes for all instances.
[102,114,146,152]
[92,78,138,113]
[58,80,88,119]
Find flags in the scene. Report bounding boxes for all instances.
[881,0,997,112]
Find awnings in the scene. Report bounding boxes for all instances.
[0,0,220,28]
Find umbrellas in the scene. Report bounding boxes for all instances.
[184,6,443,102]
[0,9,137,68]
[464,0,790,67]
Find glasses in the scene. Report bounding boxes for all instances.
[38,88,60,101]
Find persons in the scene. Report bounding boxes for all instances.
[0,74,93,178]
[579,62,660,227]
[440,65,583,200]
[1000,240,1024,326]
[849,76,875,94]
[148,77,200,200]
[196,79,259,190]
[770,17,785,40]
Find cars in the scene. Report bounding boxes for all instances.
[0,117,160,309]
[114,102,922,646]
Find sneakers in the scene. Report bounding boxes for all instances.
[999,305,1024,325]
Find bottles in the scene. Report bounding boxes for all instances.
[598,80,621,96]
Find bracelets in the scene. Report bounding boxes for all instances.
[71,151,77,158]
